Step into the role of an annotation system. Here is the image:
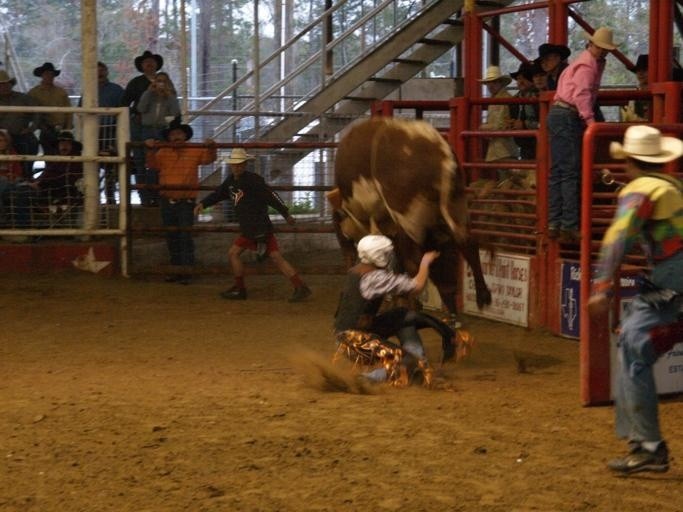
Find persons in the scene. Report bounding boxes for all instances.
[193,149,312,303]
[477,29,650,241]
[145,120,217,285]
[584,126,683,475]
[333,235,474,388]
[0,50,180,207]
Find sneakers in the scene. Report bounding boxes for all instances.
[163,272,180,284]
[547,225,561,239]
[288,282,311,304]
[177,275,200,286]
[219,285,249,300]
[560,226,581,247]
[606,438,672,478]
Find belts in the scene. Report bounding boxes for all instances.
[551,100,579,113]
[160,197,196,205]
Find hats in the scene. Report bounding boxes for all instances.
[538,42,571,58]
[54,131,83,154]
[533,57,544,66]
[608,123,683,164]
[33,62,61,78]
[583,27,616,51]
[163,119,193,142]
[474,65,513,86]
[525,60,545,83]
[223,147,257,166]
[630,54,648,74]
[0,70,18,86]
[134,50,164,73]
[510,61,528,80]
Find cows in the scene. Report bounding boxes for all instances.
[324,115,493,319]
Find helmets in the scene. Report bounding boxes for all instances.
[356,233,393,264]
